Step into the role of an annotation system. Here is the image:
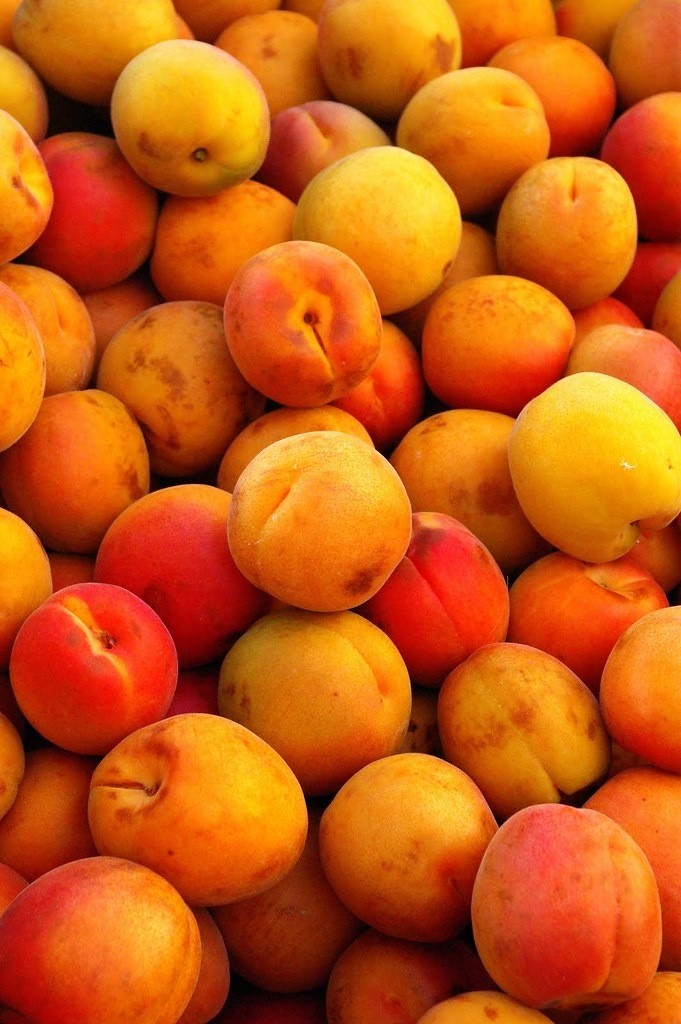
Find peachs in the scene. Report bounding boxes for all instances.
[0,1,681,1024]
